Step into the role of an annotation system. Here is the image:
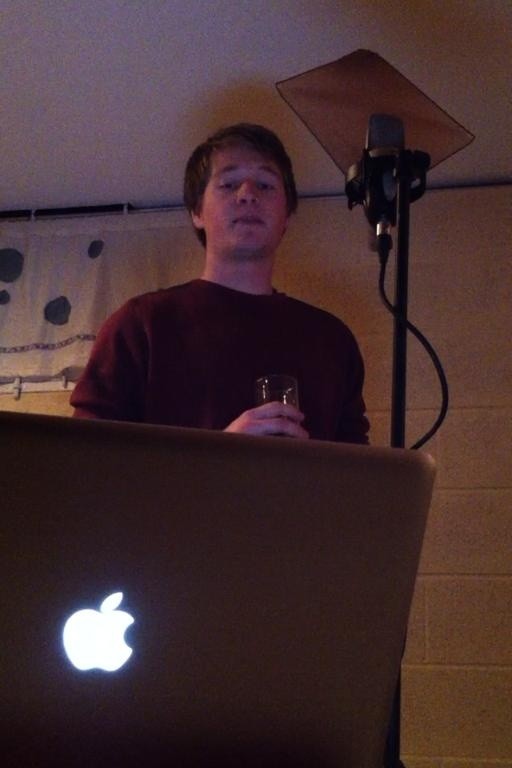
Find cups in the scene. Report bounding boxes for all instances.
[253,371,301,434]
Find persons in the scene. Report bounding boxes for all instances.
[69,117,374,448]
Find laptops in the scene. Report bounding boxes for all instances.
[0,412,437,767]
